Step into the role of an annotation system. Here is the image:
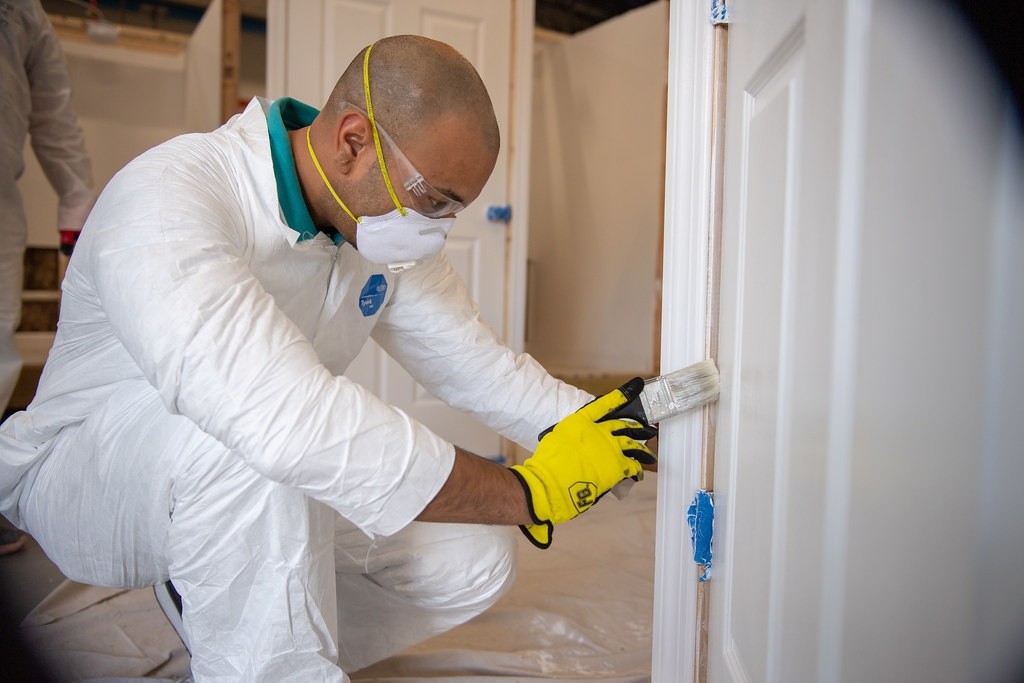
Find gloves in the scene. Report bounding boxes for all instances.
[58,230,80,256]
[506,378,659,549]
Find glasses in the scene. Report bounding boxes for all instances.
[341,101,468,218]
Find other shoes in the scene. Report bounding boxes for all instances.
[0,528,27,554]
[153,580,191,659]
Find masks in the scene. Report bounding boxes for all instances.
[356,207,457,275]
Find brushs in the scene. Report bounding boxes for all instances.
[538,359,719,451]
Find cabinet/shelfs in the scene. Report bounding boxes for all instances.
[187,1,536,474]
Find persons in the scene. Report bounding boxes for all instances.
[0,1,99,553]
[0,35,659,683]
